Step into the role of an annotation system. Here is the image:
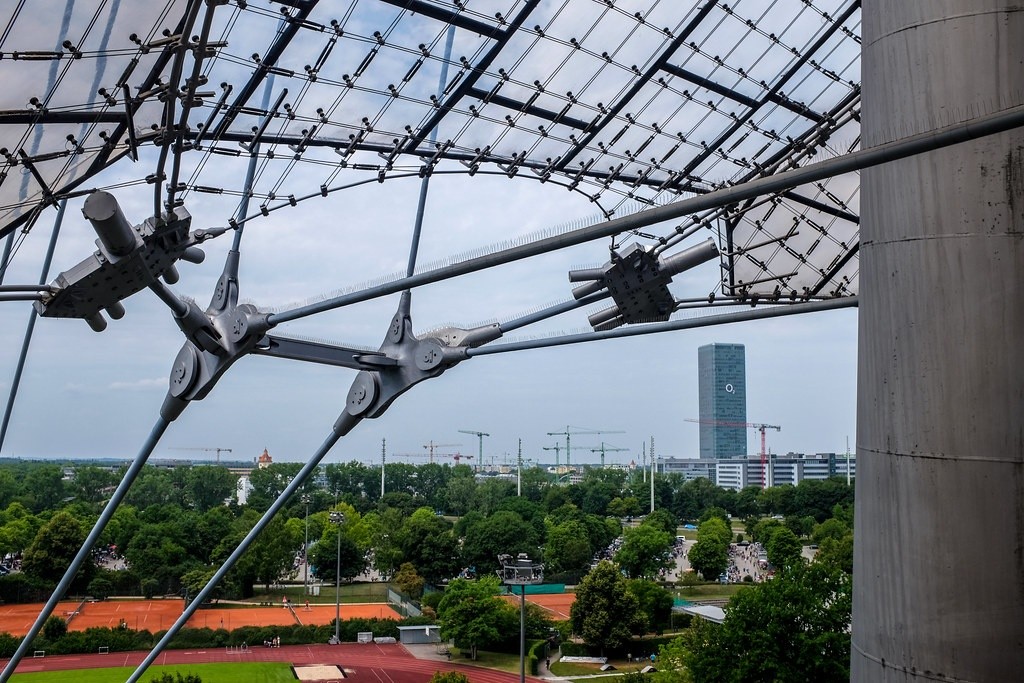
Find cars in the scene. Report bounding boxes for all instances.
[676,535,685,542]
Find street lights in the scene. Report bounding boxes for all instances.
[299,493,314,595]
[627,653,631,678]
[329,512,345,640]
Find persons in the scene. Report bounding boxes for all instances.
[657,537,688,578]
[91,543,130,572]
[264,636,280,648]
[726,542,775,583]
[120,617,127,628]
[650,653,656,667]
[362,545,390,582]
[221,617,224,628]
[546,659,550,670]
[593,544,620,562]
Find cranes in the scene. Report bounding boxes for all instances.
[458,430,489,473]
[543,442,591,483]
[168,447,232,465]
[547,425,626,485]
[683,419,780,495]
[488,455,498,465]
[392,451,474,464]
[423,440,463,464]
[590,442,630,468]
